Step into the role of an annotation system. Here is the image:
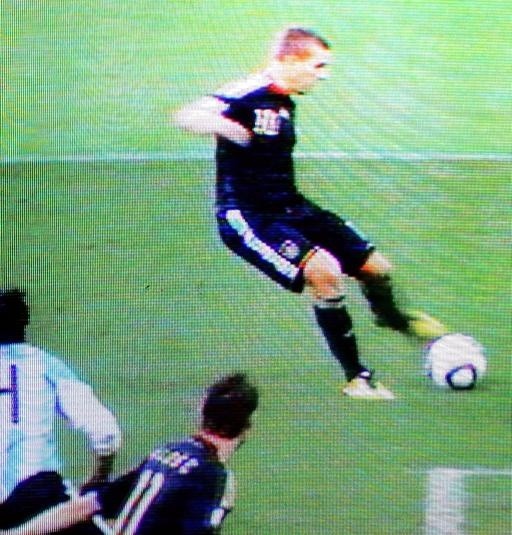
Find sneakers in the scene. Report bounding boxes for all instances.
[344,373,392,399]
[375,308,446,338]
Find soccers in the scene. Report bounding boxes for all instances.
[426,333,487,388]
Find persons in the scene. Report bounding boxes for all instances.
[1,290,120,535]
[9,372,258,535]
[171,25,447,400]
